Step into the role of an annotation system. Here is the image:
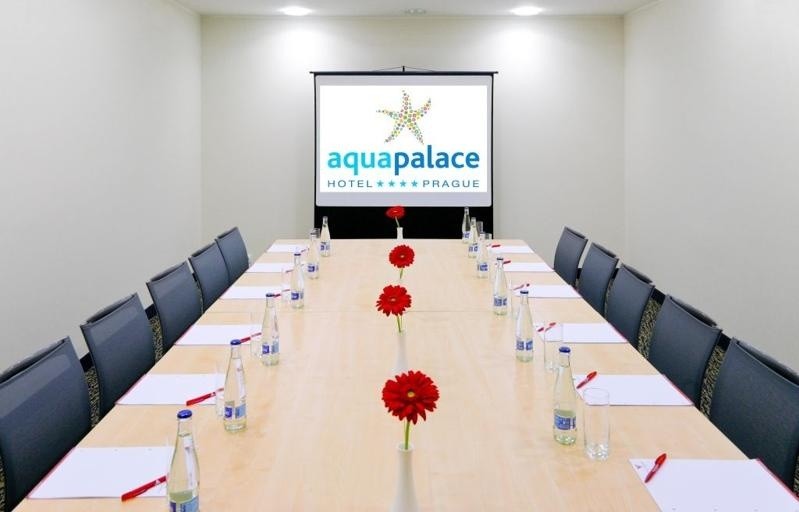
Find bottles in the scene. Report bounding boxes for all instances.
[286,217,332,305]
[222,339,251,437]
[549,343,578,445]
[257,289,279,366]
[460,206,508,315]
[513,289,535,363]
[167,409,201,512]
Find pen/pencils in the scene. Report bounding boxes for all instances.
[644,453,667,483]
[491,244,501,247]
[274,289,290,298]
[239,333,262,343]
[301,248,309,252]
[538,322,556,332]
[577,371,597,388]
[513,285,524,291]
[121,476,167,502]
[186,388,224,406]
[286,264,304,273]
[495,260,511,265]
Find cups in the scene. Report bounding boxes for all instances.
[542,320,564,373]
[250,312,262,340]
[581,386,613,460]
[213,395,225,418]
